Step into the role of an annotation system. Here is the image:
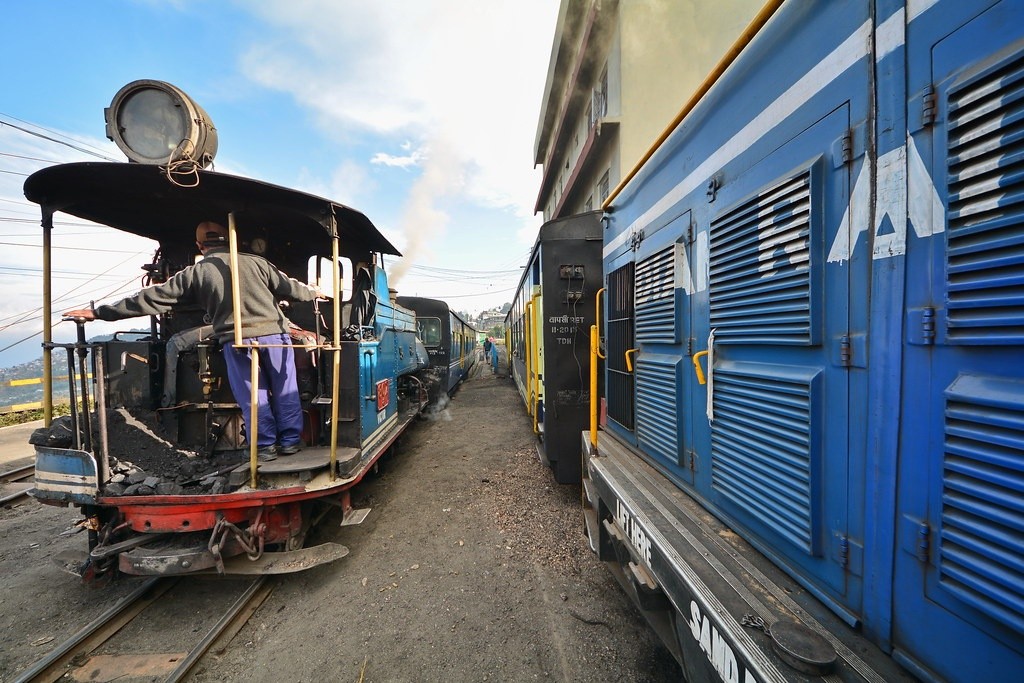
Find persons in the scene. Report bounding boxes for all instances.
[62,221,325,462]
[484,338,492,364]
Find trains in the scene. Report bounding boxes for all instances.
[23,79,476,576]
[503,0,1024,682]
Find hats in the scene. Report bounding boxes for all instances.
[196,221,229,241]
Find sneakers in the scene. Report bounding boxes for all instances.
[242,445,278,461]
[280,437,306,454]
